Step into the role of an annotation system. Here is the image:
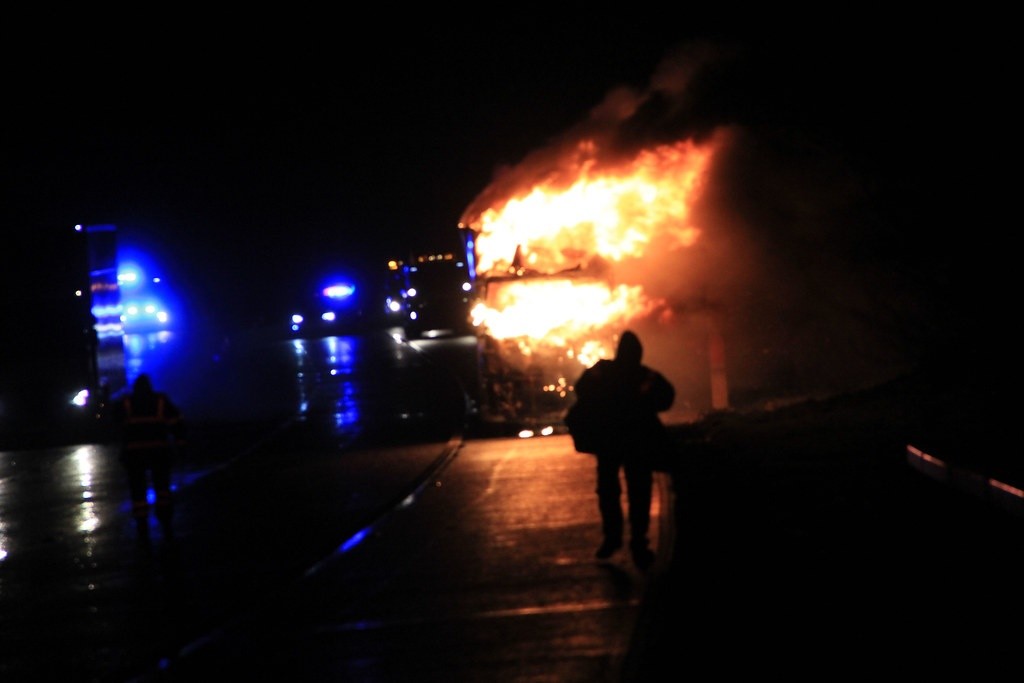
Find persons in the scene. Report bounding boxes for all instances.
[574,329,677,567]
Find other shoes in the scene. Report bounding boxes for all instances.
[596,535,622,559]
[632,549,652,565]
